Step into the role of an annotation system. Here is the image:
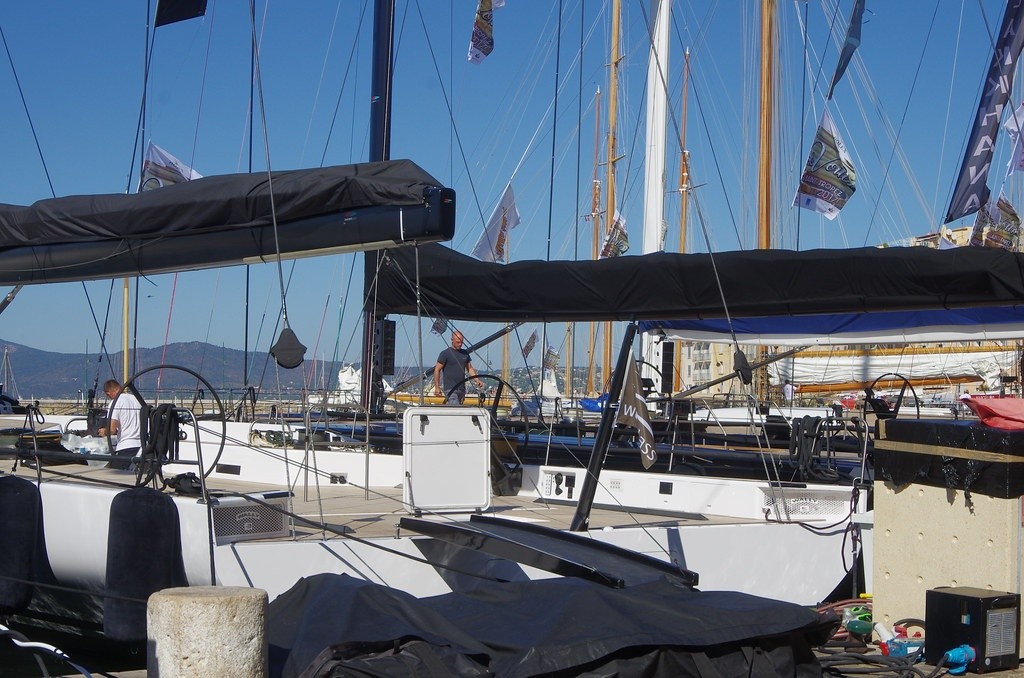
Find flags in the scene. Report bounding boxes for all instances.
[429,318,447,337]
[471,184,524,265]
[826,0,864,101]
[937,0,1024,252]
[135,138,204,194]
[543,346,561,372]
[523,328,539,358]
[153,0,207,30]
[792,107,856,221]
[598,208,629,259]
[466,0,505,66]
[615,348,658,470]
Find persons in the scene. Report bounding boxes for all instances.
[982,381,986,394]
[959,389,972,416]
[781,379,801,407]
[433,331,483,405]
[1014,381,1019,393]
[98,380,143,469]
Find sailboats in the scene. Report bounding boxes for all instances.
[0,0,1024,677]
